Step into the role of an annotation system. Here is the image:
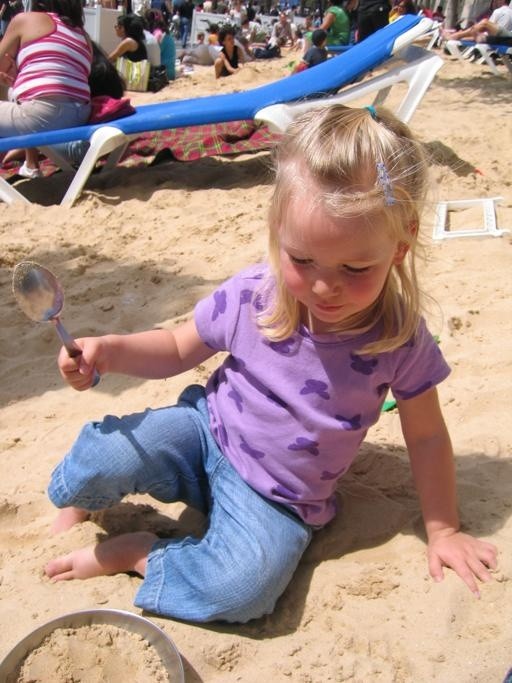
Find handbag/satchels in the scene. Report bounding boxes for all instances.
[87,95,136,124]
[149,64,169,93]
[116,57,150,92]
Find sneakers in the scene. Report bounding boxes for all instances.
[17,160,41,180]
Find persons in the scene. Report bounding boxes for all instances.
[0,0,95,177]
[0,0,512,93]
[39,101,498,624]
[1,37,128,165]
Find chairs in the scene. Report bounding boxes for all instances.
[1,15,445,207]
[447,40,512,79]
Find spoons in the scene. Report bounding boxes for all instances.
[10,260,101,390]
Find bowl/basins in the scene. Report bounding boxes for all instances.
[1,609,185,683]
